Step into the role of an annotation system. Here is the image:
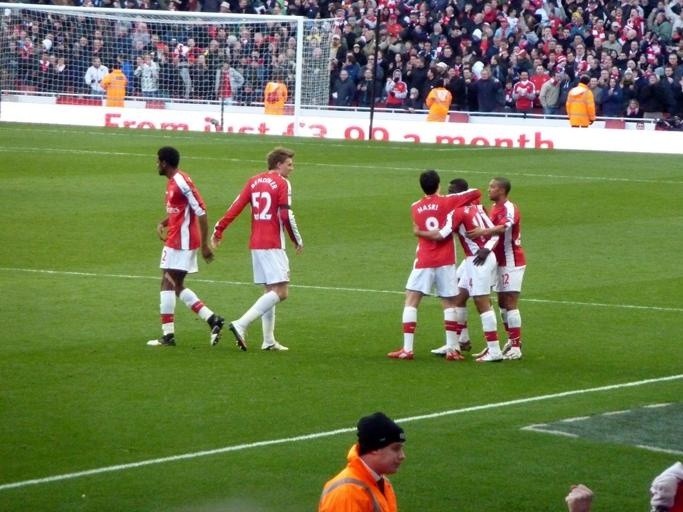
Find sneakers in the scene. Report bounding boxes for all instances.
[445,350,464,361]
[431,343,448,354]
[504,341,523,360]
[261,340,289,351]
[460,341,474,350]
[475,352,502,363]
[208,315,224,347]
[146,334,175,347]
[229,321,249,350]
[386,347,414,360]
[472,348,488,357]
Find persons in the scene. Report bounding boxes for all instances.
[210,144,304,353]
[647,461,682,512]
[465,177,526,363]
[144,144,225,347]
[409,179,502,364]
[385,168,480,362]
[459,203,500,358]
[562,483,596,512]
[316,411,407,512]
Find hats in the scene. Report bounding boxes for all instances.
[357,411,408,456]
